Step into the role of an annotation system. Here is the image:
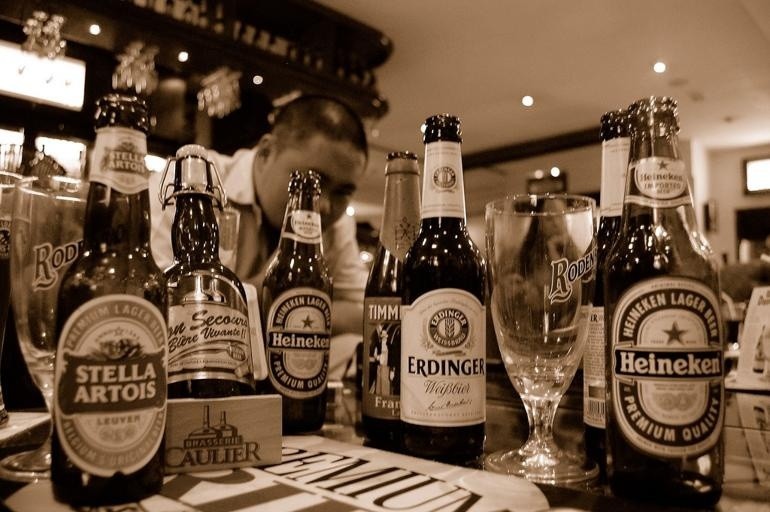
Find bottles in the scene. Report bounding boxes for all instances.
[583,94,727,510]
[162,142,257,400]
[399,113,488,466]
[361,150,423,451]
[260,167,334,436]
[49,92,171,509]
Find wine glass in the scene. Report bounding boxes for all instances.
[483,192,600,486]
[1,174,91,485]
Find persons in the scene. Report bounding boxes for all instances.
[352,218,393,291]
[513,196,581,273]
[65,91,370,340]
